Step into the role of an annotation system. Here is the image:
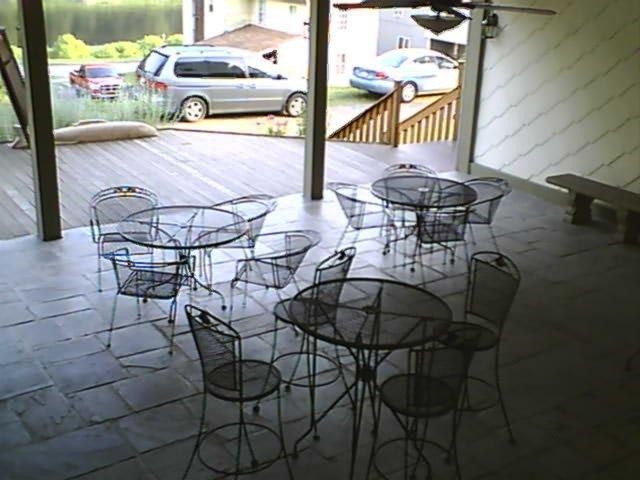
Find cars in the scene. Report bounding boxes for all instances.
[349,48,460,103]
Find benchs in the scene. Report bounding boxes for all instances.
[544,170,639,248]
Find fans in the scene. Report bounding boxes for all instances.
[332,0,558,26]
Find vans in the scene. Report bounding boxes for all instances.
[131,43,310,122]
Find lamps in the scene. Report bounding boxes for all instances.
[480,11,503,41]
[406,10,468,38]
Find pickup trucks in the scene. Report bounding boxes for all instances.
[70,62,126,102]
